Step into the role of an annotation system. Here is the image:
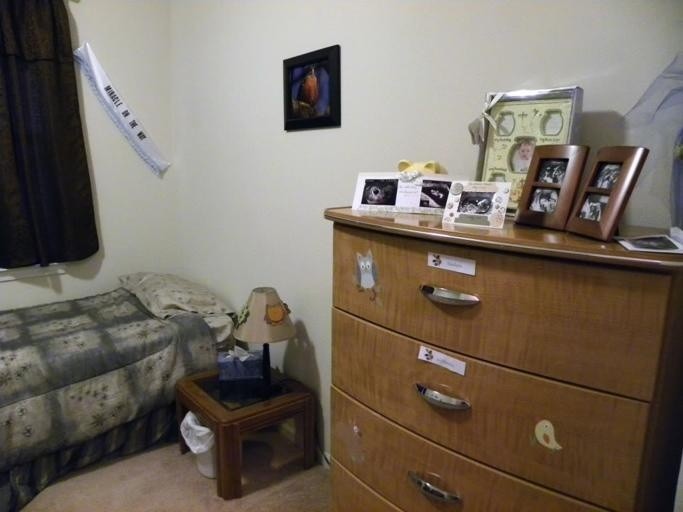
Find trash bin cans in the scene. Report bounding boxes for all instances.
[188,410,217,479]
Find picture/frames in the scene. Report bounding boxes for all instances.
[350,170,454,219]
[440,177,514,227]
[281,44,343,134]
[512,142,591,233]
[561,143,651,242]
[473,84,586,217]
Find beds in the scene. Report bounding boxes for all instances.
[0,274,239,510]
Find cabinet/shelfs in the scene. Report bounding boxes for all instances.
[320,200,680,511]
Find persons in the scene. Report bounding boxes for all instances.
[512,140,534,171]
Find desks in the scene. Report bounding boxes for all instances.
[174,366,320,499]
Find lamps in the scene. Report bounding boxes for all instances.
[231,285,300,383]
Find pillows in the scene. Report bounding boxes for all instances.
[118,269,231,321]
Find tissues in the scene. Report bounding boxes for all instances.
[215,345,263,381]
